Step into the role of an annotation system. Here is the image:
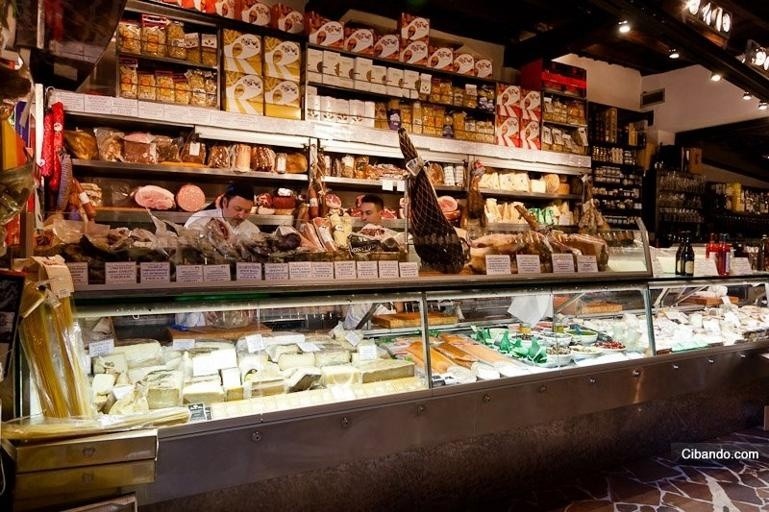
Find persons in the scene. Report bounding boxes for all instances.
[180,180,266,250]
[357,194,386,226]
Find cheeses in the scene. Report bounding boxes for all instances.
[84,331,414,415]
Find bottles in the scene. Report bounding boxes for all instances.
[747,234,769,271]
[705,232,731,276]
[675,230,694,277]
[593,145,641,228]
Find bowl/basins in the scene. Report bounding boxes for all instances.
[476,320,626,366]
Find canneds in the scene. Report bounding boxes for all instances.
[590,146,642,224]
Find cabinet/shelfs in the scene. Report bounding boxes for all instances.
[26,0,769,505]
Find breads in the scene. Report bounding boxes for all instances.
[64,127,409,180]
